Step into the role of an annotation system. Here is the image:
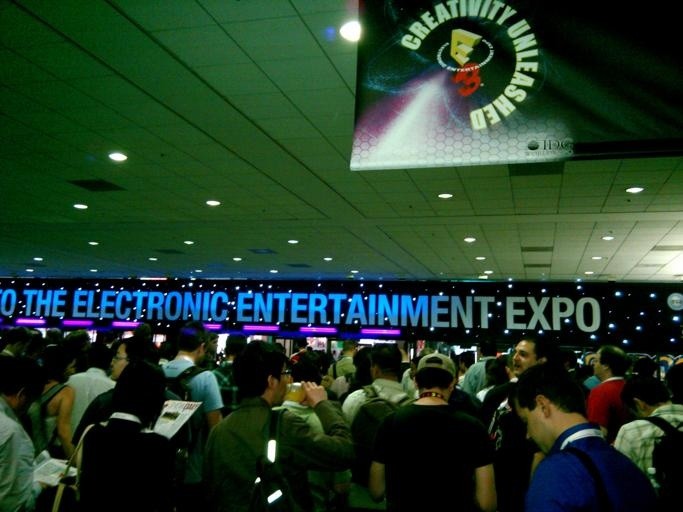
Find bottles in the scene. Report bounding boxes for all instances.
[176,447,188,478]
[283,382,327,406]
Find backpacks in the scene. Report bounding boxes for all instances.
[23,382,68,453]
[162,366,208,448]
[642,411,682,512]
[352,384,414,455]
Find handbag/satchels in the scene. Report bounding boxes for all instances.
[254,406,314,512]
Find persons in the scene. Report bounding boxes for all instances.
[206,335,354,512]
[341,339,496,510]
[483,339,682,512]
[0,323,224,512]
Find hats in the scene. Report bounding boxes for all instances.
[416,352,456,377]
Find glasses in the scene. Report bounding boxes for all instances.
[281,369,294,377]
[112,356,128,361]
